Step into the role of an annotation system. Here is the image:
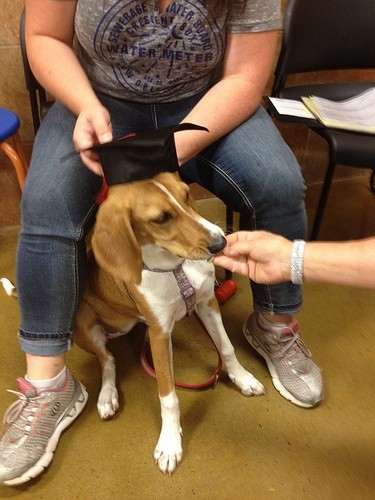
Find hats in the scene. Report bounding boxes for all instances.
[60,122,210,203]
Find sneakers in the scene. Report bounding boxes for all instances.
[0,365,89,486]
[242,311,324,409]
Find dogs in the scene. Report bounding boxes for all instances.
[0,169,266,477]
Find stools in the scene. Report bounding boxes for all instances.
[0,108,28,199]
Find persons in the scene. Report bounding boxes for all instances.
[212,230,374,289]
[0,0,325,488]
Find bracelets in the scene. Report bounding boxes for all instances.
[290,238,306,285]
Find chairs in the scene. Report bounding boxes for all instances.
[269,0,375,241]
[19,8,235,281]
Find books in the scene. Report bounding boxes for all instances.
[262,86,375,135]
